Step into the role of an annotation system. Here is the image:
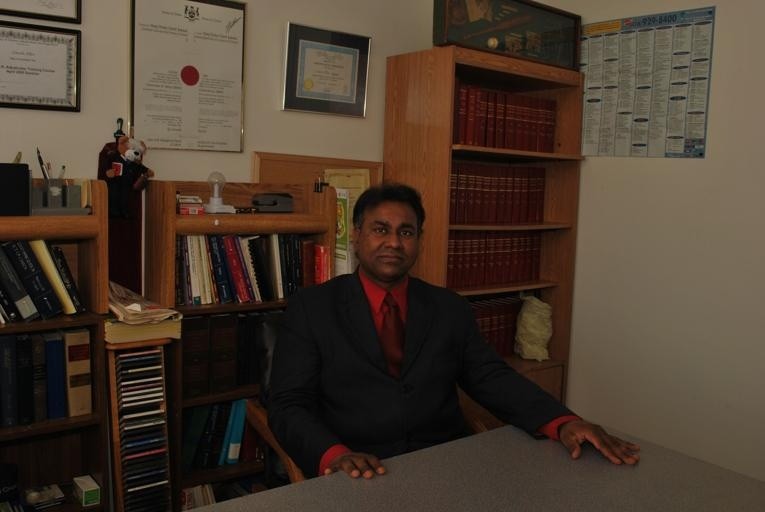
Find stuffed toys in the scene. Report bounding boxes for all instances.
[106,136,155,192]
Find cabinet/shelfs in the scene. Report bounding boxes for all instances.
[0,179,116,512]
[143,179,338,510]
[383,48,587,430]
[106,338,173,512]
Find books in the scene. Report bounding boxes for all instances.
[444,76,560,361]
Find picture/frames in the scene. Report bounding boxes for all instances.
[282,22,371,120]
[0,0,88,112]
[129,0,245,154]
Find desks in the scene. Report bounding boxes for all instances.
[192,415,765,512]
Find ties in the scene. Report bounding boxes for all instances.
[378,291,406,380]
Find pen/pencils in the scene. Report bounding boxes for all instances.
[13,152,22,163]
[36,146,66,179]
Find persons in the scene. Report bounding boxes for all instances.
[268,176,640,479]
